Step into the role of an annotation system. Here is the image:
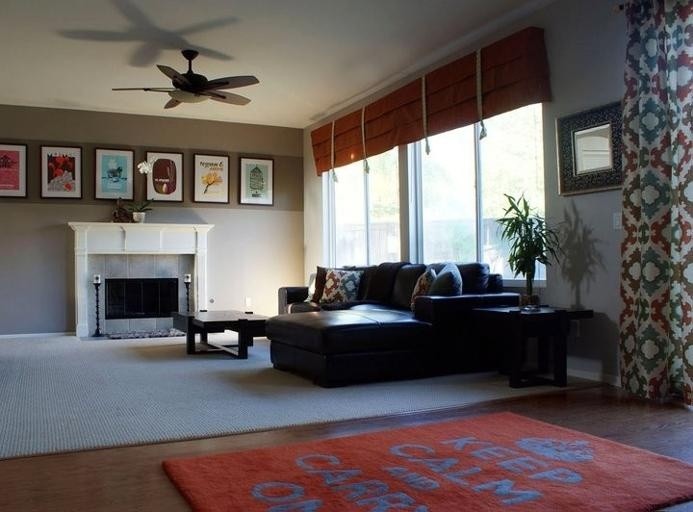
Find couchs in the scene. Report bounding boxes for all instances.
[265,273,521,389]
[279,261,426,314]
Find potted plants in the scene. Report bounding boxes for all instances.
[126,199,154,223]
[496,195,567,312]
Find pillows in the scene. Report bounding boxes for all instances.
[409,268,437,311]
[426,264,489,295]
[362,261,409,305]
[391,264,427,310]
[341,267,376,301]
[320,269,363,304]
[310,267,355,302]
[428,262,461,297]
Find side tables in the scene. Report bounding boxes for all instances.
[472,304,594,388]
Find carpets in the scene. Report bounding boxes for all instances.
[0,329,603,460]
[160,412,693,512]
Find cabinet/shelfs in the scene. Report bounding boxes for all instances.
[69,222,215,338]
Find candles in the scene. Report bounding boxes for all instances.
[93,274,100,283]
[184,274,191,282]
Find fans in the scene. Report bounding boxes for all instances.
[112,50,260,109]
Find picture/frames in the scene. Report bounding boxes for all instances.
[238,157,274,206]
[144,151,184,202]
[194,154,231,204]
[94,148,135,202]
[40,144,82,199]
[555,100,623,197]
[0,141,28,198]
